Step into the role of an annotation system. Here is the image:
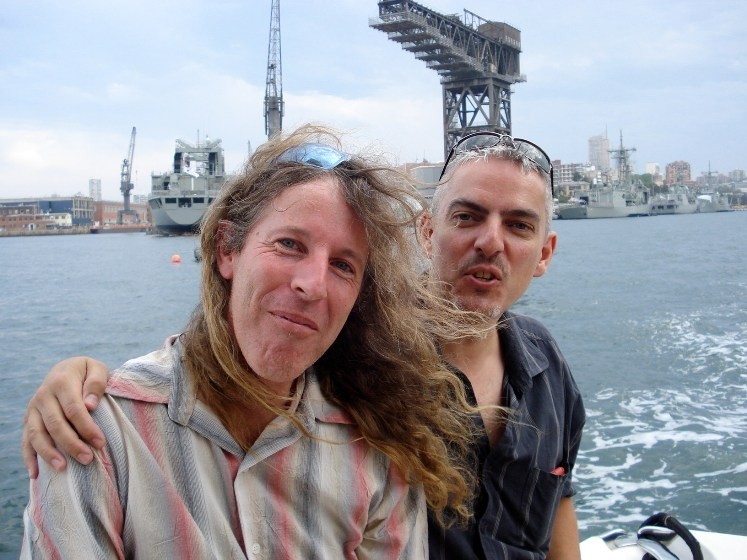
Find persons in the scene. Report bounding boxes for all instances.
[19,124,541,560]
[22,130,586,560]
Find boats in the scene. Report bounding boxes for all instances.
[563,127,735,221]
[146,127,226,237]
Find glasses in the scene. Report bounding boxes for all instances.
[438,131,557,200]
[275,143,374,174]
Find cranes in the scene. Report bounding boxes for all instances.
[117,124,141,226]
